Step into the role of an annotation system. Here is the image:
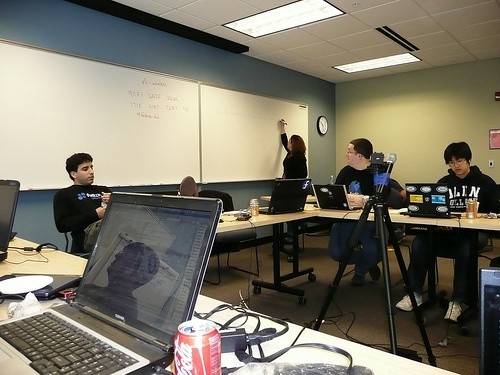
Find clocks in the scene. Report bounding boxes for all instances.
[317,116,329,135]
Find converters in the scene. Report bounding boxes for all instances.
[219,327,247,353]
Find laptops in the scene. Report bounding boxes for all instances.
[406,183,466,219]
[258,178,312,214]
[312,185,363,211]
[0,180,20,262]
[0,192,223,375]
[479,267,500,375]
[0,274,82,301]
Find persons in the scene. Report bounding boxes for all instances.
[279,119,308,179]
[396,142,500,321]
[80,241,160,320]
[53,153,111,254]
[329,139,407,286]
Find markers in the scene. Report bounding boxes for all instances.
[281,119,287,125]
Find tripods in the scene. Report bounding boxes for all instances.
[313,193,438,368]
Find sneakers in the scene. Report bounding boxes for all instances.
[396,292,422,312]
[444,301,463,322]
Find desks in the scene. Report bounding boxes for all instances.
[0,193,500,375]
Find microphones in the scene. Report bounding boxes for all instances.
[371,153,397,194]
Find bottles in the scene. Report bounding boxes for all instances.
[329,175,335,185]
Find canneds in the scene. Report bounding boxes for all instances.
[466,200,477,219]
[173,319,221,375]
[250,198,259,216]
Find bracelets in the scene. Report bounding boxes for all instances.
[364,195,369,202]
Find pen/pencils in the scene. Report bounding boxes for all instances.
[99,192,103,196]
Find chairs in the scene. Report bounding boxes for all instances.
[198,190,259,286]
[428,233,494,324]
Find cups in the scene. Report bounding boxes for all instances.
[304,204,314,211]
[239,208,248,217]
[248,205,258,221]
[466,201,479,218]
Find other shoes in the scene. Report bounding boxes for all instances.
[351,264,381,284]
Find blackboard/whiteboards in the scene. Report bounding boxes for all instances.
[199,81,310,184]
[0,38,201,192]
